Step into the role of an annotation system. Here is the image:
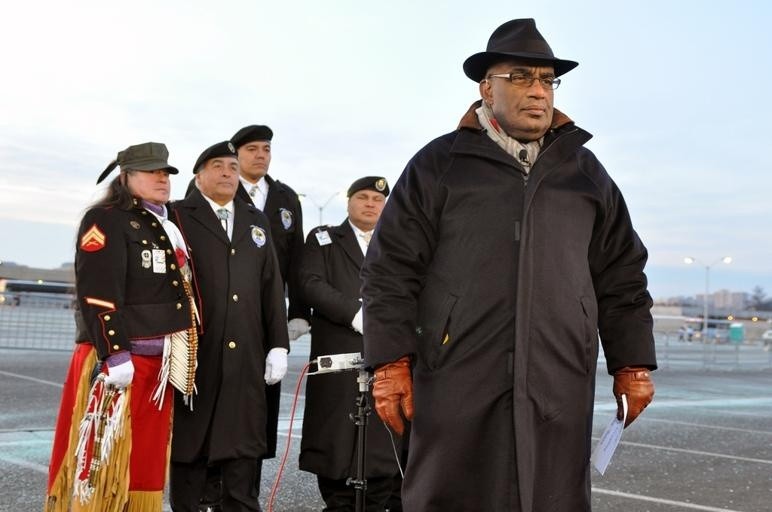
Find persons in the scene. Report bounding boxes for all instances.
[357,16,658,511]
[172,140,292,512]
[43,141,210,511]
[183,124,305,512]
[289,174,418,512]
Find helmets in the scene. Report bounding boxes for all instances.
[193,141,238,174]
[118,142,179,175]
[348,176,389,197]
[229,125,273,148]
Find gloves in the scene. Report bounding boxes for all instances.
[288,319,312,339]
[105,360,135,388]
[351,306,363,335]
[372,357,414,435]
[264,348,289,385]
[612,368,655,429]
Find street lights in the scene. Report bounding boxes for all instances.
[683,253,736,344]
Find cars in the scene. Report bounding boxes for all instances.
[761,330,772,349]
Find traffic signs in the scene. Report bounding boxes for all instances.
[296,192,344,228]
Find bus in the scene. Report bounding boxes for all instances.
[1,278,79,312]
[680,317,734,344]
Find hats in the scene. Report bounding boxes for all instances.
[463,19,578,82]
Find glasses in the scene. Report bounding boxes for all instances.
[487,73,561,90]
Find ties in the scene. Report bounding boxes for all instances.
[218,209,228,218]
